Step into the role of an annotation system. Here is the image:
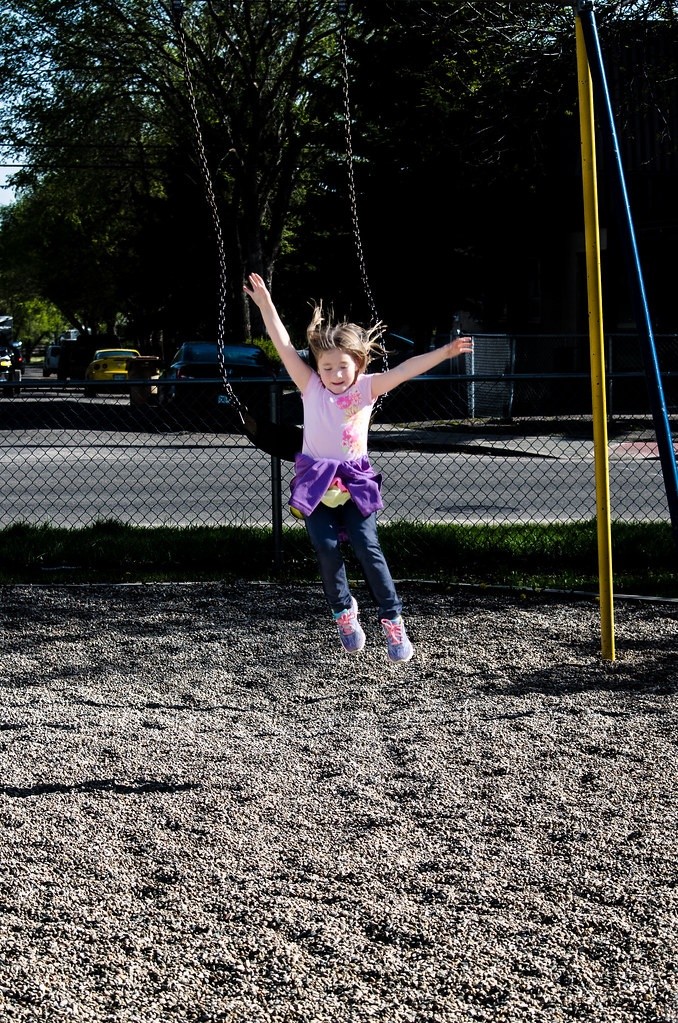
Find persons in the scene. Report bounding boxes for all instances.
[243,273,475,664]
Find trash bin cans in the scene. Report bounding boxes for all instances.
[124,355,164,407]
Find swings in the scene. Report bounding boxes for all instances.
[174,7,389,463]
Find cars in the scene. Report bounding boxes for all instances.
[84,348,143,397]
[154,341,282,418]
[42,344,61,377]
[0,344,25,376]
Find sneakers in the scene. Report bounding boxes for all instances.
[380,614,413,664]
[331,596,366,654]
